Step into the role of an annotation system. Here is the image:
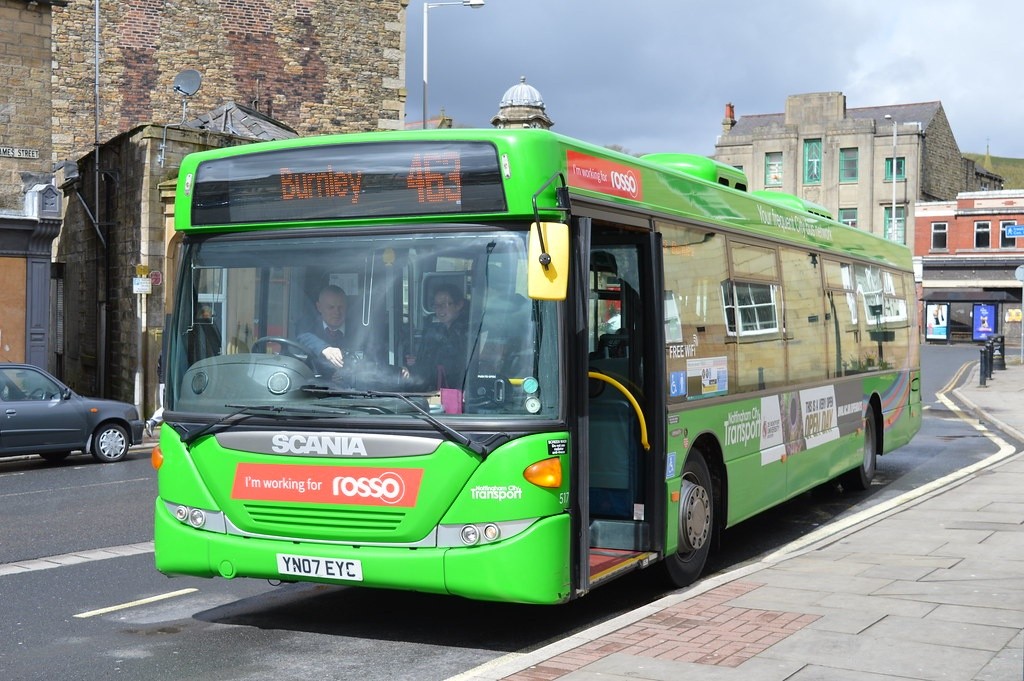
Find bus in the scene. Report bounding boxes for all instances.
[154,128,922,617]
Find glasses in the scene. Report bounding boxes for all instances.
[432,299,463,309]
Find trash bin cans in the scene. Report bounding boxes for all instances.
[988,335,1005,370]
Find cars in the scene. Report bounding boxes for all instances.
[0,363,145,464]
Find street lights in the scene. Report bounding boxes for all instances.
[422,0,485,128]
[885,114,896,242]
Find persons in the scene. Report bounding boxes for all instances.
[145,349,165,436]
[402,284,469,393]
[298,283,376,381]
[607,308,621,334]
